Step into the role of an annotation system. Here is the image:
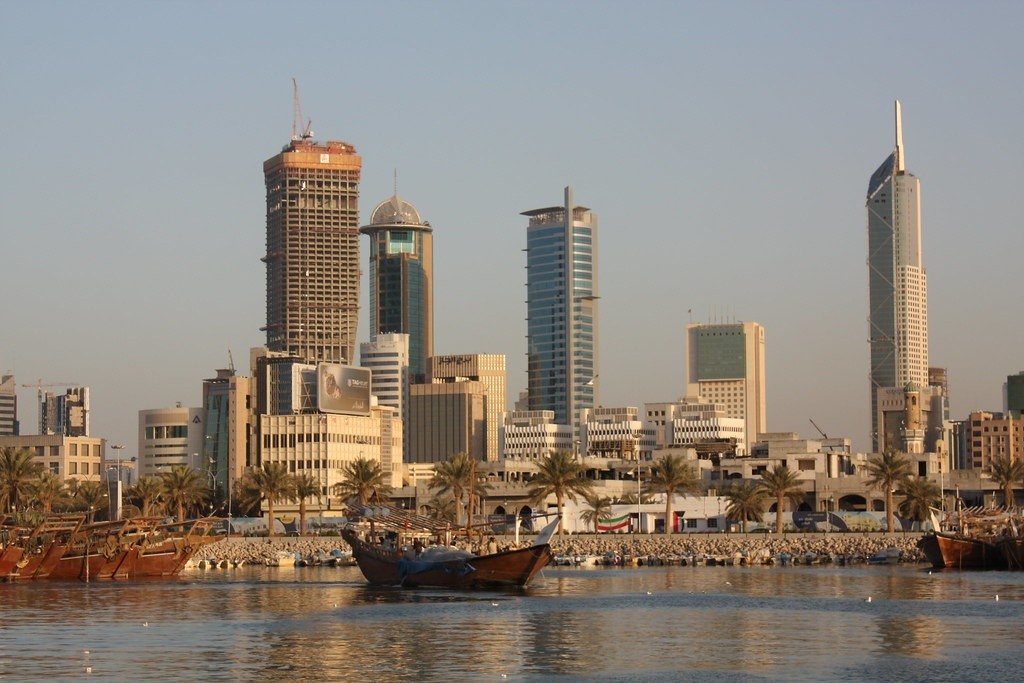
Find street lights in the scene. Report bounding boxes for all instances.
[193,465,235,499]
[110,442,126,520]
[190,453,199,465]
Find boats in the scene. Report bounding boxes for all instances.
[994,514,1024,571]
[338,510,566,594]
[917,482,984,571]
[1,507,229,585]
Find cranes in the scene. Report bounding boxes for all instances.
[21,377,79,435]
[226,347,236,377]
[289,76,316,140]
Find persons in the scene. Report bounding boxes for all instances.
[379,535,500,559]
